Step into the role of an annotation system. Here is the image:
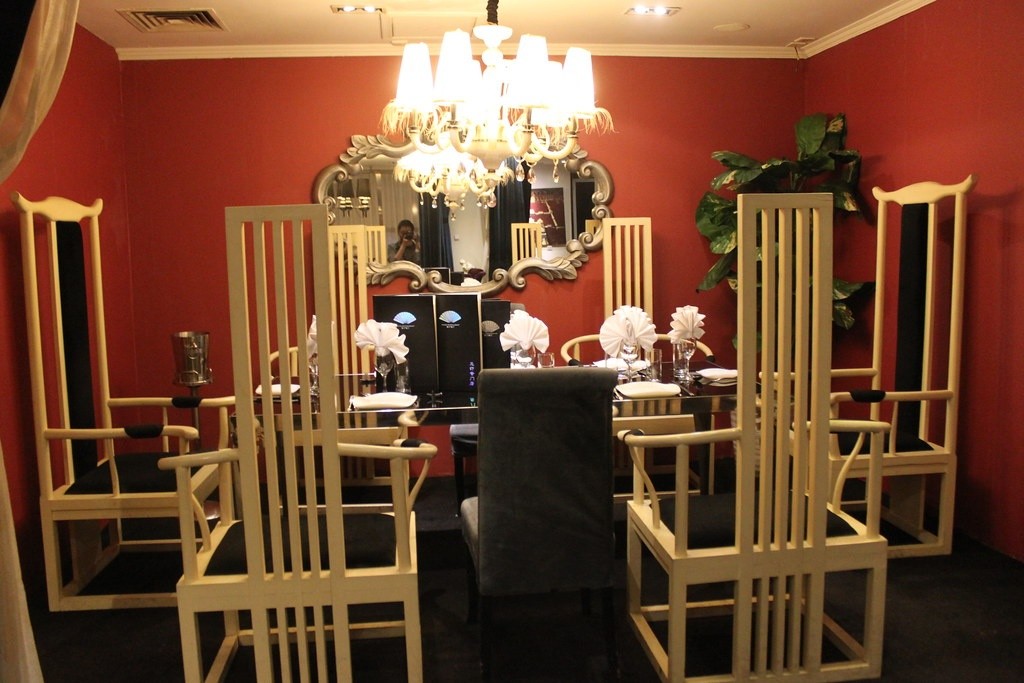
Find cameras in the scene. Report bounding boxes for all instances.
[405,231,414,240]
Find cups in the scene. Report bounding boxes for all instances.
[393,360,412,395]
[645,350,662,383]
[538,352,555,368]
[673,344,689,378]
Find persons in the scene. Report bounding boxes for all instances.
[387,220,420,262]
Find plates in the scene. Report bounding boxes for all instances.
[616,381,681,398]
[255,384,300,395]
[353,392,418,409]
[696,368,738,378]
[593,357,651,371]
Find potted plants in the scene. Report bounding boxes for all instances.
[694,108,875,475]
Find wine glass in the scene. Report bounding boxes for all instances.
[310,365,320,395]
[623,347,637,383]
[681,339,696,381]
[373,354,393,392]
[515,345,536,369]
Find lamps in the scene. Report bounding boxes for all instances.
[383,1,596,211]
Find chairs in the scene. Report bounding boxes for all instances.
[510,221,542,269]
[364,225,388,265]
[12,174,993,683]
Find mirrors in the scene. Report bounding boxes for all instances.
[312,133,611,301]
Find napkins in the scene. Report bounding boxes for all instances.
[500,309,549,354]
[355,320,409,363]
[667,305,707,343]
[599,305,658,357]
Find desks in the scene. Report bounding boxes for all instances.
[230,361,797,414]
